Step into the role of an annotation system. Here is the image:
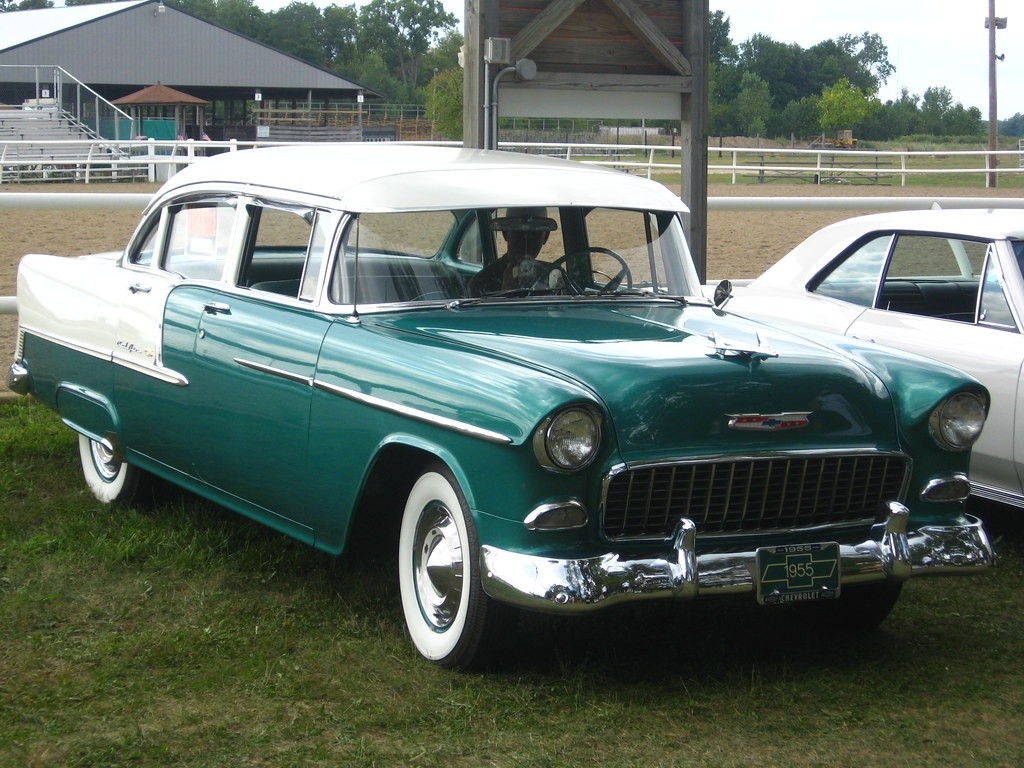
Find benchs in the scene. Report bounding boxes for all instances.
[171,256,469,306]
[822,281,1014,326]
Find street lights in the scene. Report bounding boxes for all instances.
[431,67,439,130]
[984,0,1008,187]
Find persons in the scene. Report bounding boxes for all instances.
[463,207,567,299]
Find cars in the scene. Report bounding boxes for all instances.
[4,140,1001,671]
[637,207,1024,509]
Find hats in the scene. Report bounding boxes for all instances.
[489,207,557,231]
